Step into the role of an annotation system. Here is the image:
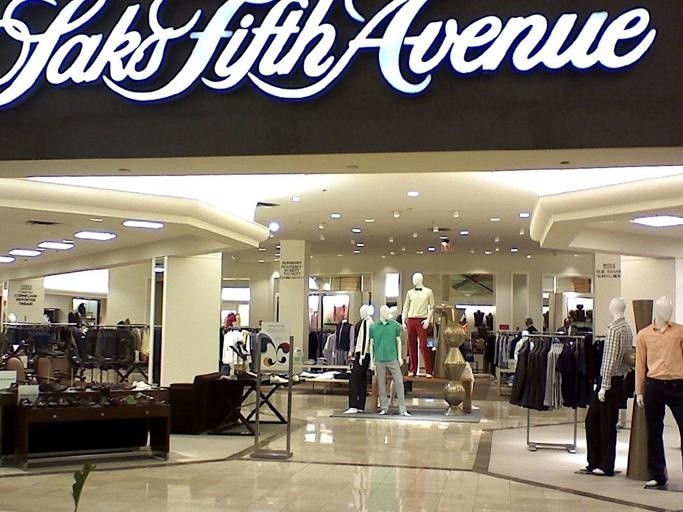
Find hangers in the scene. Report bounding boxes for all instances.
[86,327,141,340]
[1,323,78,340]
[486,332,605,359]
[224,326,261,338]
[309,330,336,339]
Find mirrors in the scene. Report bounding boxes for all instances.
[73,298,98,329]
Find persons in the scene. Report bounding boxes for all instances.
[402,272,435,378]
[634,297,683,486]
[332,304,411,417]
[473,310,493,328]
[525,318,538,334]
[557,317,578,343]
[585,298,633,476]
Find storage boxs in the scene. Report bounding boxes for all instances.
[331,276,360,291]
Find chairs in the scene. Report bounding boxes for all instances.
[4,356,86,384]
[170,372,222,434]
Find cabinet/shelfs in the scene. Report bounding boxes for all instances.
[575,322,593,331]
[522,335,585,453]
[303,365,353,393]
[207,378,305,436]
[470,331,479,349]
[324,323,339,349]
[0,387,170,470]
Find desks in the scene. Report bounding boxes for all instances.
[86,359,148,382]
[473,354,485,373]
[495,366,516,396]
[372,376,474,413]
